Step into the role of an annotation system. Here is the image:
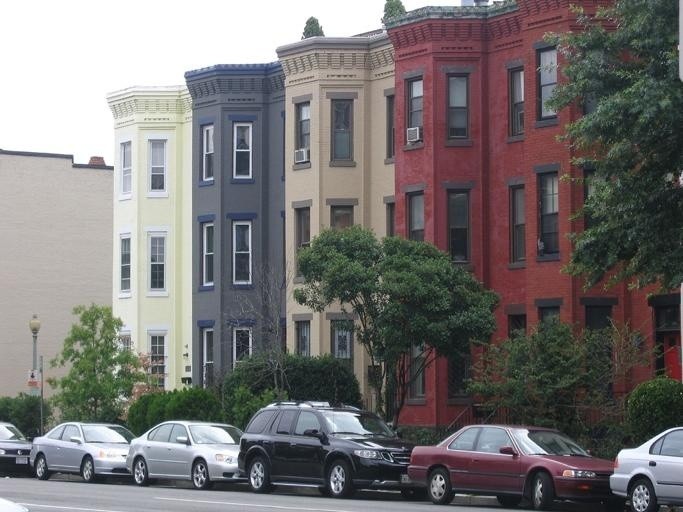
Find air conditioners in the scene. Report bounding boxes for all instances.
[294,148,310,164]
[406,125,422,142]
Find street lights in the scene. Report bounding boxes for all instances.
[28,315,41,372]
